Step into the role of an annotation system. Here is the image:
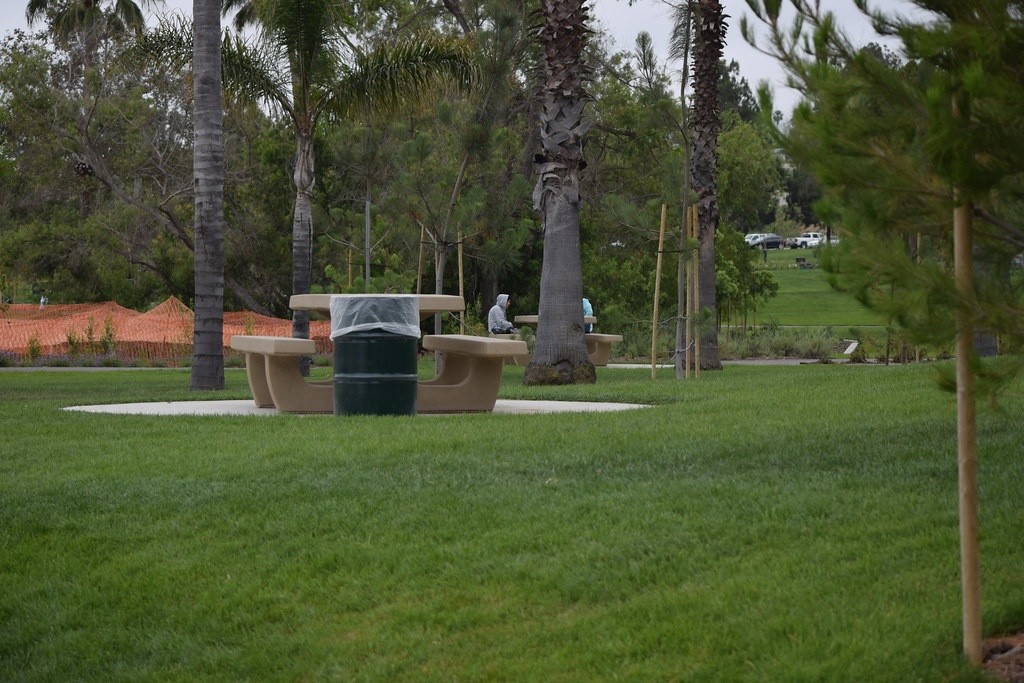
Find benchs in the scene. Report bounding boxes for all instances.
[489,334,533,366]
[228,334,317,412]
[421,333,529,411]
[584,333,623,365]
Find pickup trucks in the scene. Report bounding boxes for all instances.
[787,232,825,249]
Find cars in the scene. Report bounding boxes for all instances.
[750,236,787,250]
[808,235,838,248]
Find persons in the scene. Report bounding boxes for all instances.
[583,298,594,333]
[488,292,516,334]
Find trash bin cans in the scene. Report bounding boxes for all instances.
[331,294,420,415]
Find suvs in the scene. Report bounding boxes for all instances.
[744,232,776,246]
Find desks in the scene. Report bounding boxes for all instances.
[288,293,465,413]
[513,315,599,324]
